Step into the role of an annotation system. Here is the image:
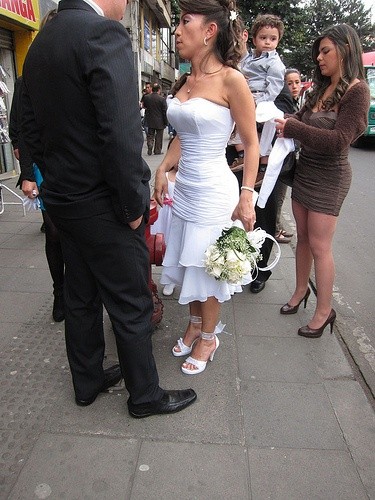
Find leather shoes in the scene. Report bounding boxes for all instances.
[128,389,197,418]
[251,280,265,293]
[76,364,121,406]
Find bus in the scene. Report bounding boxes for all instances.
[350,52,375,149]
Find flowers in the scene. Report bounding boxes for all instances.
[201,220,266,285]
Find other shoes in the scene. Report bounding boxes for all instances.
[255,172,264,188]
[278,229,293,237]
[230,158,244,172]
[275,233,291,243]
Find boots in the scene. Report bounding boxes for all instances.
[53,284,67,322]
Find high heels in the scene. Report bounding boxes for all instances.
[182,332,219,374]
[298,309,336,338]
[172,316,202,356]
[280,287,310,314]
